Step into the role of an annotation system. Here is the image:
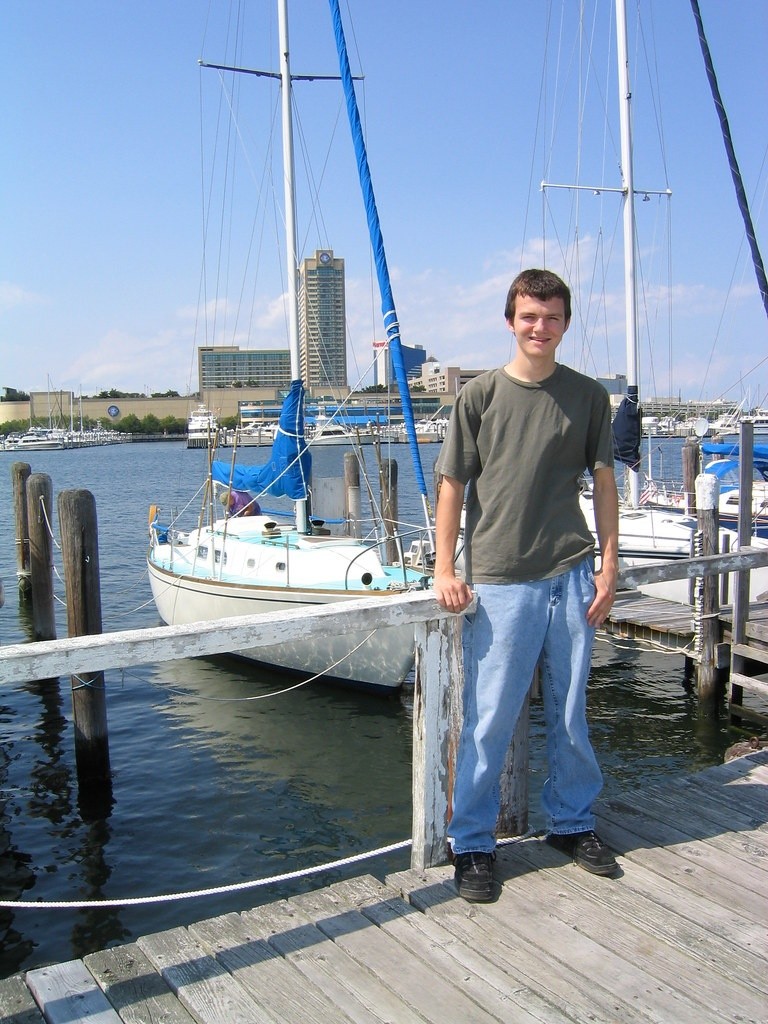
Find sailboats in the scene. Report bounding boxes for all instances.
[143,0,452,689]
[459,1,768,610]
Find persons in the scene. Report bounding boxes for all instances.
[220,491,261,517]
[433,268,620,902]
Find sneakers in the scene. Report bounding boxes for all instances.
[546,830,624,874]
[452,850,497,900]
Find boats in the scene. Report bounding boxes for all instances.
[589,442,768,539]
[0,373,127,451]
[182,363,768,449]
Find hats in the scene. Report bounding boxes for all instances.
[220,492,233,505]
